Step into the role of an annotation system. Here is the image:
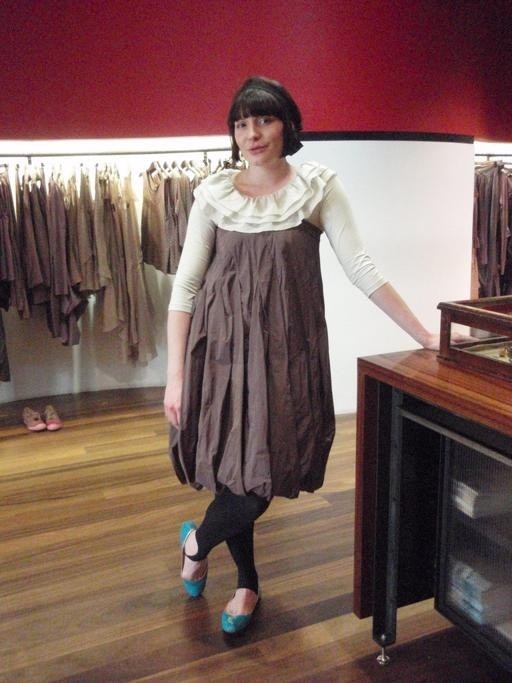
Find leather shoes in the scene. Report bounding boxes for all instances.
[221,591,259,634]
[179,520,208,600]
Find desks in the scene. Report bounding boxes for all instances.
[343,344,512,666]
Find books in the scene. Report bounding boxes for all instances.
[449,472,512,646]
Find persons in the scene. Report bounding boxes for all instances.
[162,75,482,637]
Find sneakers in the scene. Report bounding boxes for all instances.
[22,405,64,430]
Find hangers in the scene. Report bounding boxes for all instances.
[0,152,242,212]
